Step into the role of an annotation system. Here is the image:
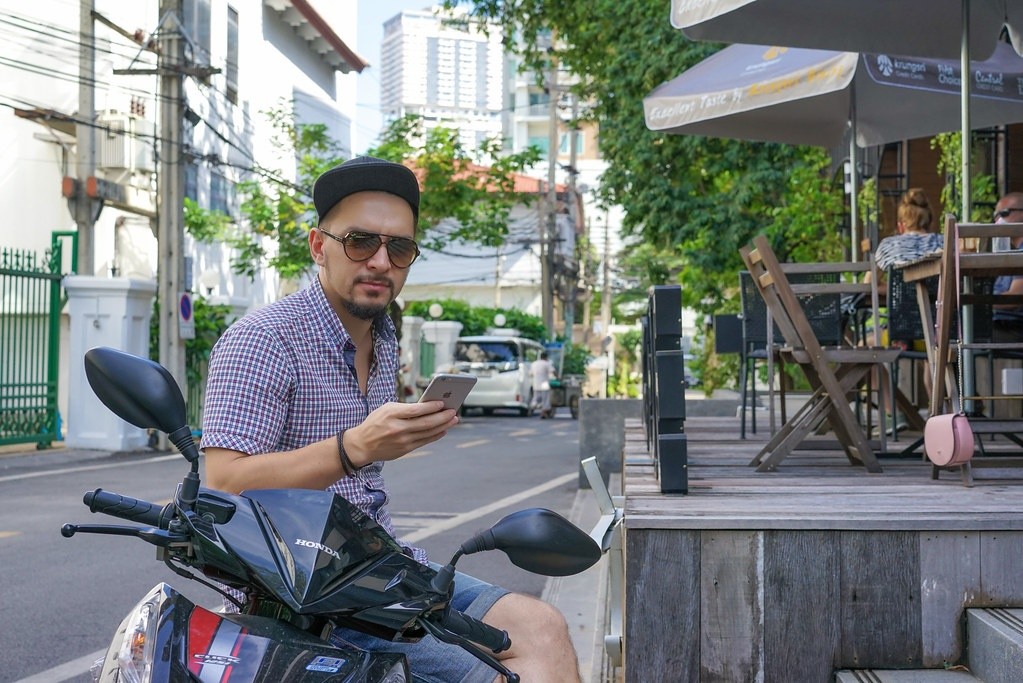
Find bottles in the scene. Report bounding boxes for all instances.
[993,218,1011,251]
[815,249,830,262]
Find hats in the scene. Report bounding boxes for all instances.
[313,154,421,225]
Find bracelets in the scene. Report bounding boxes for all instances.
[337,429,373,480]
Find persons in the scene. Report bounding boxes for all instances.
[200,160,581,683]
[991,192,1023,320]
[527,353,558,419]
[858,188,945,415]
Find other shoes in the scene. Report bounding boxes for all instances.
[974,393,986,417]
[918,408,932,419]
[871,412,910,436]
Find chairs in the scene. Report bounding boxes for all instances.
[740,236,905,473]
[886,265,960,440]
[926,215,1023,487]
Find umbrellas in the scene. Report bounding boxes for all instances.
[644,42,1023,283]
[670,0,1023,413]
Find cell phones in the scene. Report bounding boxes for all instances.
[416,373,478,419]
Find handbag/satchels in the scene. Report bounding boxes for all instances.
[924,412,974,468]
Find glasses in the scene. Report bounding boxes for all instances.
[992,207,1023,220]
[318,230,421,269]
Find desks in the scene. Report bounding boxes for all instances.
[854,291,889,442]
[891,249,1023,468]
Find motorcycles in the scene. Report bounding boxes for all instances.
[59,347,602,683]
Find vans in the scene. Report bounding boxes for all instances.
[452,336,554,419]
